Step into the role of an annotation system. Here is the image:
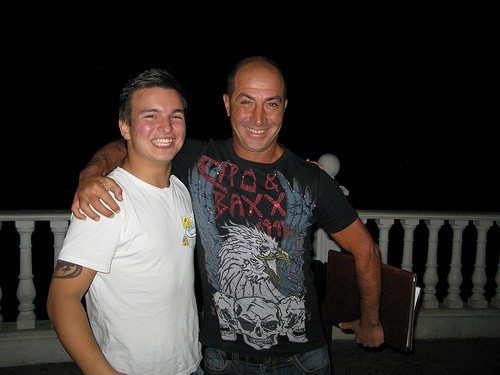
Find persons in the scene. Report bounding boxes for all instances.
[69,55,386,375]
[47,68,207,375]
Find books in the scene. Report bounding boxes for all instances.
[321,250,416,352]
